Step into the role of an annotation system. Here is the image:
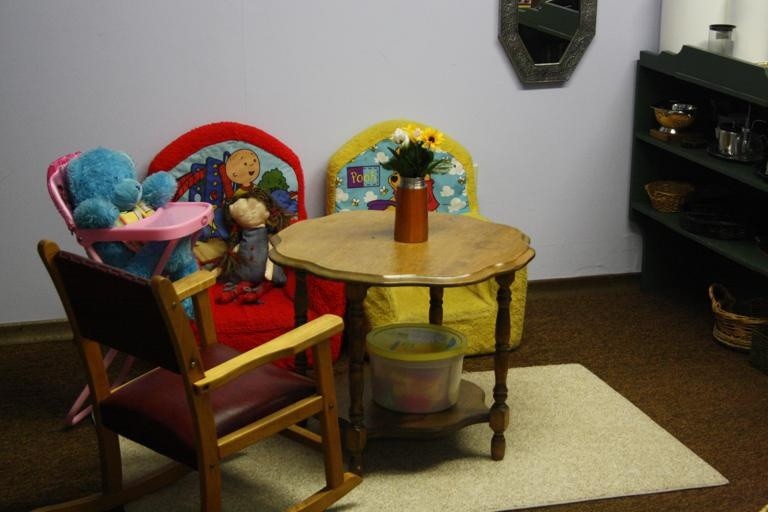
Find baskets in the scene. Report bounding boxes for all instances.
[645,181,695,212]
[708,283,767,350]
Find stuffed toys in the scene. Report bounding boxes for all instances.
[215,187,285,305]
[64,146,202,320]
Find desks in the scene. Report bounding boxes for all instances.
[267,205,536,476]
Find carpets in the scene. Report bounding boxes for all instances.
[116,362,731,512]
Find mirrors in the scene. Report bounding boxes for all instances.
[498,0,598,86]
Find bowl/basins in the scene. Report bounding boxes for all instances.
[649,99,697,128]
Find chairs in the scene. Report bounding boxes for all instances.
[326,119,529,357]
[29,239,363,512]
[149,120,346,371]
[48,156,216,427]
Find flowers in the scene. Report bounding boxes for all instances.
[379,126,456,179]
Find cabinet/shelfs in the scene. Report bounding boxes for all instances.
[627,44,768,372]
[517,0,580,63]
